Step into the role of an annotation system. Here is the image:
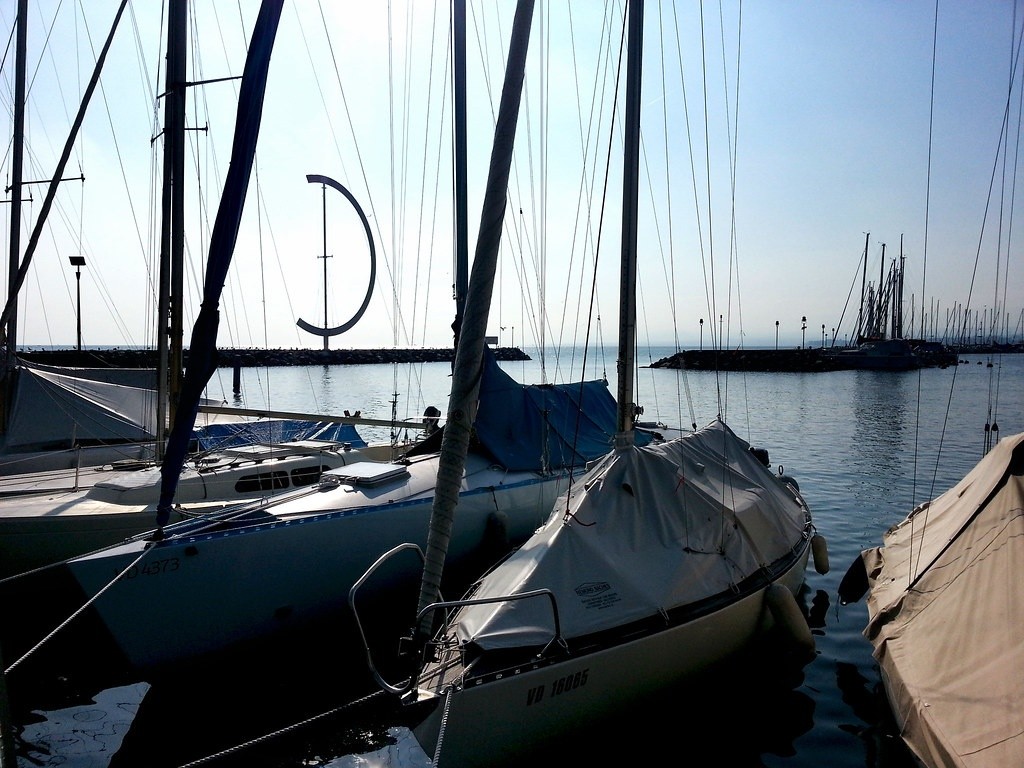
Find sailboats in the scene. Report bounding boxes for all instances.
[0,0,1024,768]
